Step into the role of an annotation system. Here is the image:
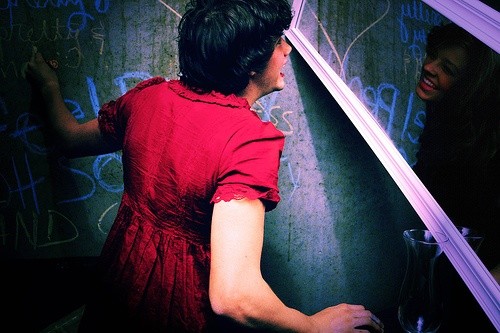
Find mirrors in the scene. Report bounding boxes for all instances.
[290,0,500,299]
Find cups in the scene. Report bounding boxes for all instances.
[397,229,451,333]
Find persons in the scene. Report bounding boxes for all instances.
[22,1,387,333]
[416,21,500,285]
